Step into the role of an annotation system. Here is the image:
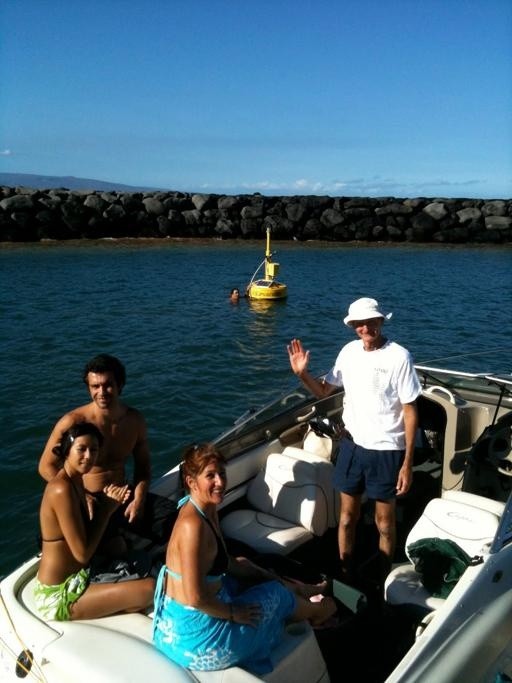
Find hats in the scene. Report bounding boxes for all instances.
[343,297,386,328]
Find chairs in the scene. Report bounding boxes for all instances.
[384,487,506,611]
[220,445,342,557]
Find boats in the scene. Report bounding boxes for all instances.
[0,347,512,679]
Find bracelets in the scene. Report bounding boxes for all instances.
[229,604,233,621]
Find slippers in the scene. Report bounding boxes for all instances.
[320,595,346,628]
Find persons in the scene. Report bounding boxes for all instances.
[230,288,239,299]
[33,421,157,622]
[36,354,180,581]
[152,444,337,676]
[286,295,424,573]
